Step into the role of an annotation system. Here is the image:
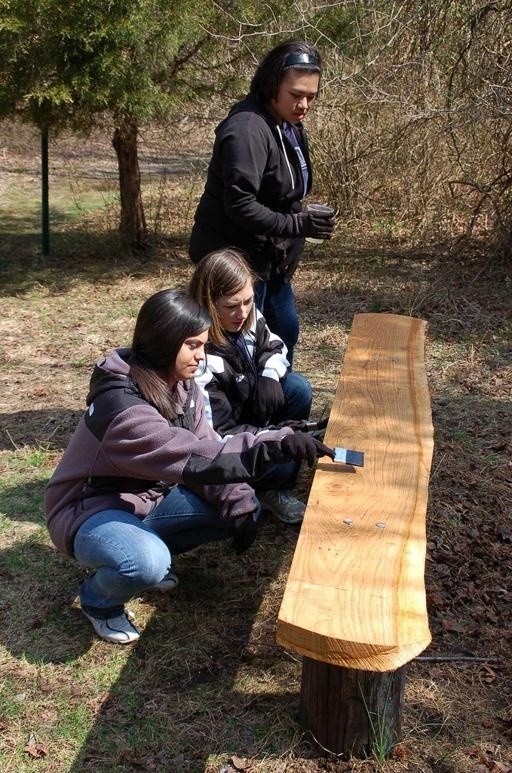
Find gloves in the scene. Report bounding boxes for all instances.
[230,512,259,553]
[253,378,286,415]
[281,430,336,468]
[275,234,306,279]
[295,206,336,240]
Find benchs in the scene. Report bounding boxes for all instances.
[273,311,438,756]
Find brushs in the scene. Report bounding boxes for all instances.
[316,447,365,467]
[301,417,329,432]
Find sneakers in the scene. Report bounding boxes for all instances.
[156,571,181,595]
[255,484,307,525]
[81,601,141,646]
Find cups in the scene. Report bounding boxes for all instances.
[305,204,334,244]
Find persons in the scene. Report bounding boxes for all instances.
[186,248,315,526]
[186,35,335,372]
[44,287,336,646]
